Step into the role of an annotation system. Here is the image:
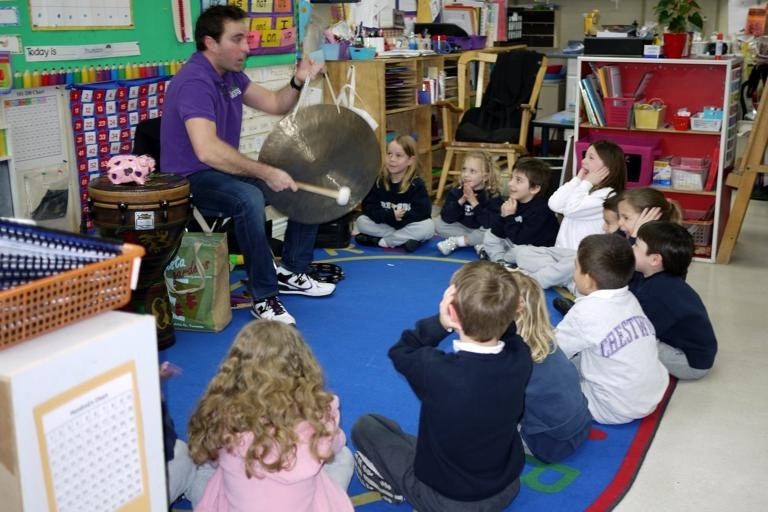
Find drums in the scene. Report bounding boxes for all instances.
[86,173,190,352]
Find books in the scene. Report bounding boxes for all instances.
[596,27,637,39]
[578,66,625,128]
[508,15,522,40]
[419,78,439,105]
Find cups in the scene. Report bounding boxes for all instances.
[664,33,686,59]
[407,30,447,54]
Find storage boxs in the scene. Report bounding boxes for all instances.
[575,137,660,191]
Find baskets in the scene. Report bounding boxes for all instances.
[0,217,149,353]
[681,217,714,248]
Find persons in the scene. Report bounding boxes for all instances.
[186,319,355,512]
[433,149,504,258]
[160,5,337,328]
[600,189,627,236]
[496,140,628,291]
[351,260,534,512]
[159,367,189,510]
[551,234,670,426]
[631,220,718,382]
[354,135,436,254]
[483,157,561,264]
[553,185,687,317]
[503,268,595,464]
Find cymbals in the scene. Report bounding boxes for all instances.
[256,104,382,224]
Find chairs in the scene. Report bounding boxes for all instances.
[433,50,549,208]
[131,119,291,292]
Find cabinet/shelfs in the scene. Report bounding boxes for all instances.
[571,54,745,263]
[323,44,523,195]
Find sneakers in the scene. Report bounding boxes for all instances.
[354,232,390,249]
[553,296,575,317]
[474,244,519,272]
[437,236,457,256]
[275,266,337,298]
[403,239,422,253]
[250,296,297,328]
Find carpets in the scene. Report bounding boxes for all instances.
[157,223,684,512]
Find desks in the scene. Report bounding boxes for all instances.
[529,111,578,143]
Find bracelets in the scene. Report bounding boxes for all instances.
[290,76,303,93]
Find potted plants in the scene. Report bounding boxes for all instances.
[654,0,706,58]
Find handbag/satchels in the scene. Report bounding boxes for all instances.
[163,231,234,334]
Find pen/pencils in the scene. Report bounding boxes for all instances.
[715,33,725,59]
[14,58,187,89]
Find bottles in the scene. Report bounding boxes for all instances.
[652,33,661,45]
[714,31,723,59]
